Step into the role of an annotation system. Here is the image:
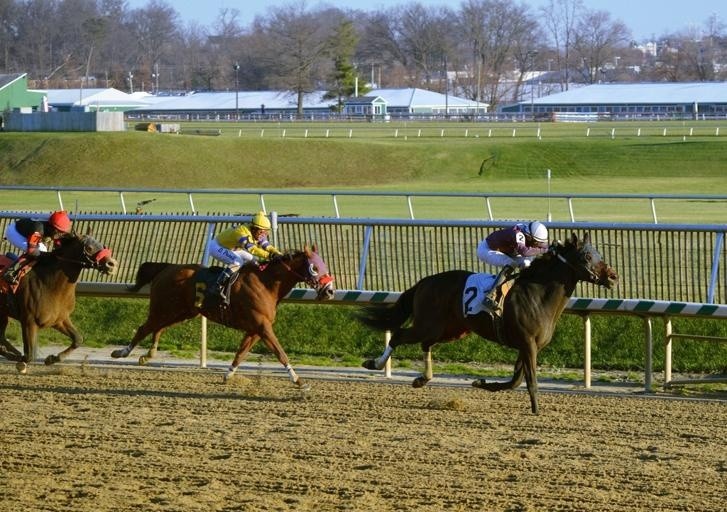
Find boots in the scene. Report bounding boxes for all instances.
[207,267,234,300]
[483,264,515,314]
[3,253,40,285]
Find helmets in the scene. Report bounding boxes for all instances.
[250,211,271,230]
[522,221,549,243]
[48,211,71,234]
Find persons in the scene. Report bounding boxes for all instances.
[209,211,282,302]
[2,209,70,285]
[475,220,549,311]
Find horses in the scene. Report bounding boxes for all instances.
[350,231,621,416]
[0,225,118,375]
[111,242,335,394]
